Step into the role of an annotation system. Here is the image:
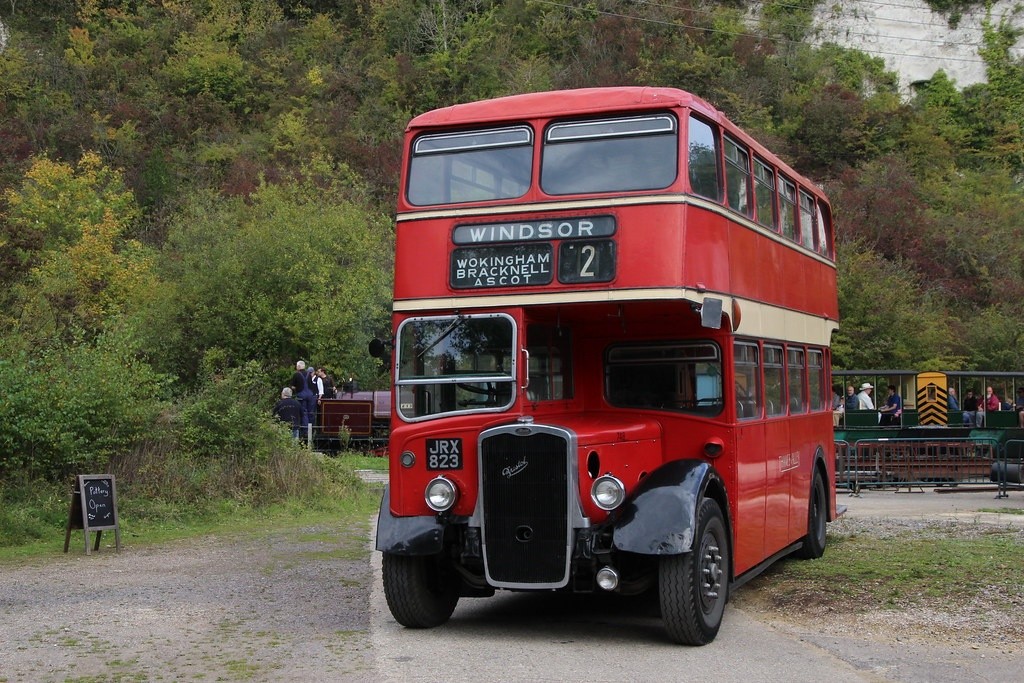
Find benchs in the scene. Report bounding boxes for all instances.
[847,407,917,428]
[947,410,1019,428]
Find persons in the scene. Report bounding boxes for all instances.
[272,361,335,438]
[947,387,1024,429]
[831,383,900,429]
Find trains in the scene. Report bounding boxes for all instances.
[278,368,1023,458]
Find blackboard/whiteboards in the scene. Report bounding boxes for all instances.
[78,476,119,531]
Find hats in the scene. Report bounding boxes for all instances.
[860,383,873,390]
[283,388,292,396]
[887,385,896,391]
[308,367,314,372]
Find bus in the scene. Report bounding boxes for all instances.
[375,88,841,647]
